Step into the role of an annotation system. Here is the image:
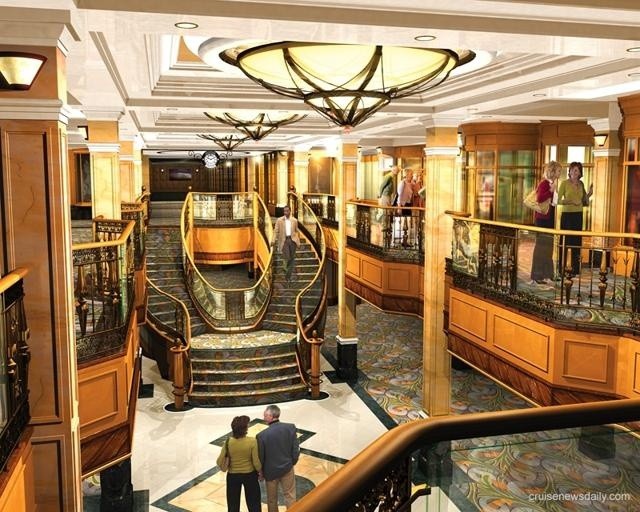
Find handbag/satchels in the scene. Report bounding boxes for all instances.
[221,457,229,472]
[582,192,589,206]
[523,190,551,215]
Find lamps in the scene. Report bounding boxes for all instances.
[230,36,463,130]
[196,133,250,153]
[592,134,607,146]
[0,51,47,90]
[206,109,309,142]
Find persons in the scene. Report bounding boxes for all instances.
[255,405,301,512]
[376,164,426,247]
[557,161,594,275]
[215,415,264,512]
[530,161,563,285]
[269,205,302,284]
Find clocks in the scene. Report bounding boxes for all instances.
[201,150,219,168]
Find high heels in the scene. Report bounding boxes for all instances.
[546,277,561,281]
[533,279,546,284]
